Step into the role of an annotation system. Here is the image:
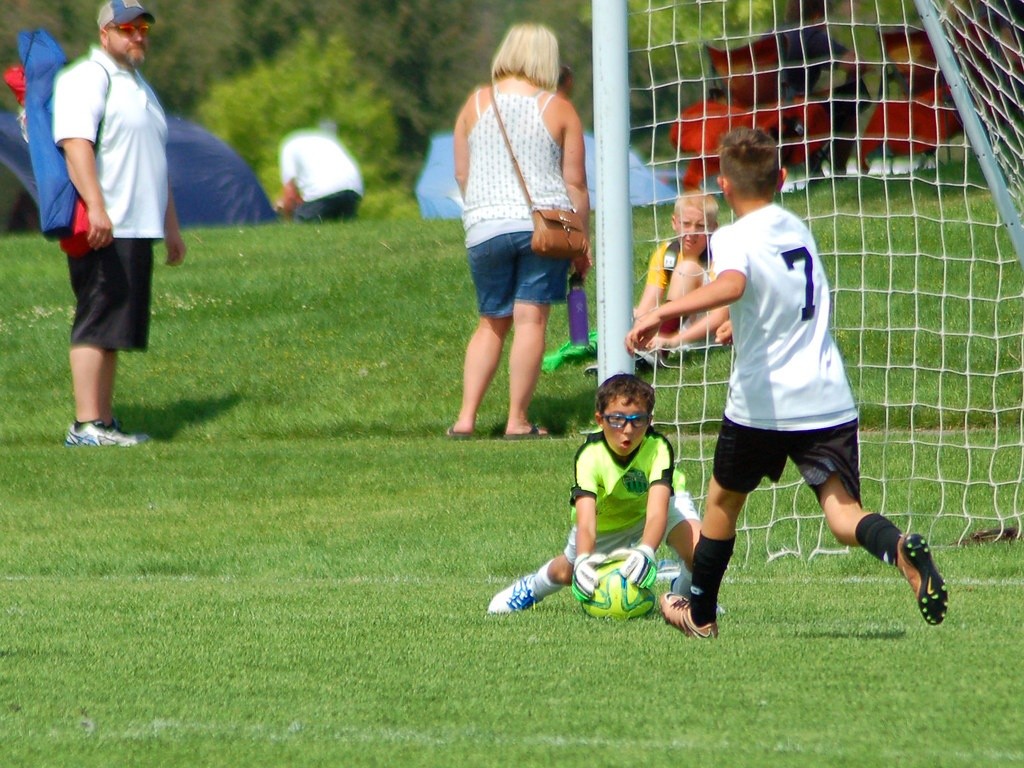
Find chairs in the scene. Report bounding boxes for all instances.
[670,12,1024,191]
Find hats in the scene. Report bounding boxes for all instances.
[96,0,155,32]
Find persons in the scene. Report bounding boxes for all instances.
[624,127,950,640]
[762,0,870,181]
[486,375,728,616]
[944,0,1021,87]
[275,136,365,223]
[445,23,591,438]
[635,191,731,373]
[50,1,189,448]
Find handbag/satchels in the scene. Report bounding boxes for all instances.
[530,209,588,259]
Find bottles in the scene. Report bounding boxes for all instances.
[568,272,588,346]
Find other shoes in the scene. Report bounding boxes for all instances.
[585,365,597,376]
[635,350,666,369]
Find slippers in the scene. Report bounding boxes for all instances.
[503,425,548,438]
[447,426,473,437]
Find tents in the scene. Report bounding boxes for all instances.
[160,115,279,225]
[418,131,681,217]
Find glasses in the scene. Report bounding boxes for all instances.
[602,413,650,428]
[105,25,151,38]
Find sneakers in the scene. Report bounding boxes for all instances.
[893,533,948,625]
[660,593,718,639]
[64,424,138,447]
[487,574,543,614]
[670,578,725,614]
[108,418,149,441]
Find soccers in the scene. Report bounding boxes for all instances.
[581,557,659,620]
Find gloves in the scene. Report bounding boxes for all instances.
[608,545,657,588]
[572,553,607,602]
